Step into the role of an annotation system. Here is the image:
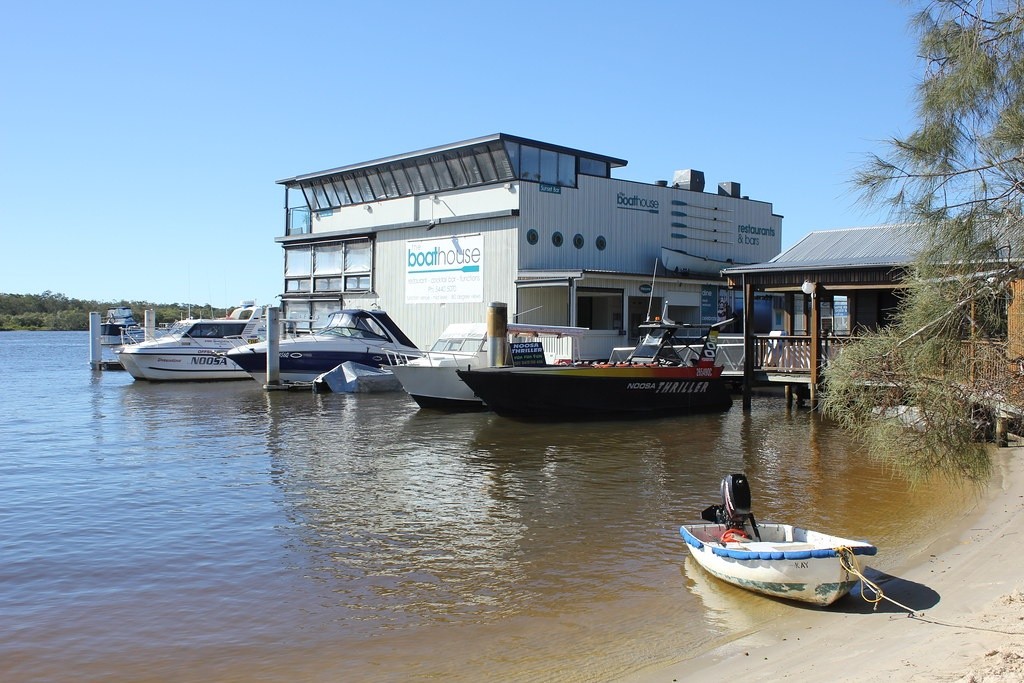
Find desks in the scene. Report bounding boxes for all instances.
[639,323,711,367]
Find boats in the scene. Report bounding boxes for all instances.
[678,472,876,608]
[101,305,180,367]
[377,320,589,412]
[210,302,421,393]
[112,301,287,383]
[456,301,735,417]
